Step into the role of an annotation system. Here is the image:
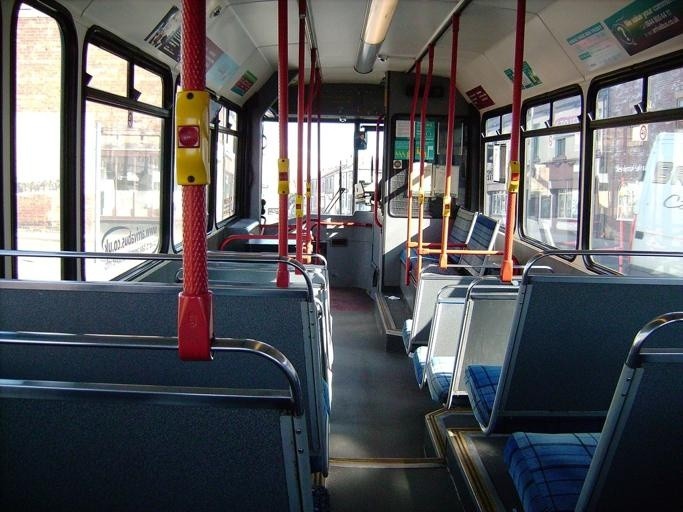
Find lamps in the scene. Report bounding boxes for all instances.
[353,0,398,76]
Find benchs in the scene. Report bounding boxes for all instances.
[1,208,331,512]
[400,207,683,511]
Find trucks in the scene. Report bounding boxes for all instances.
[627,132,683,279]
